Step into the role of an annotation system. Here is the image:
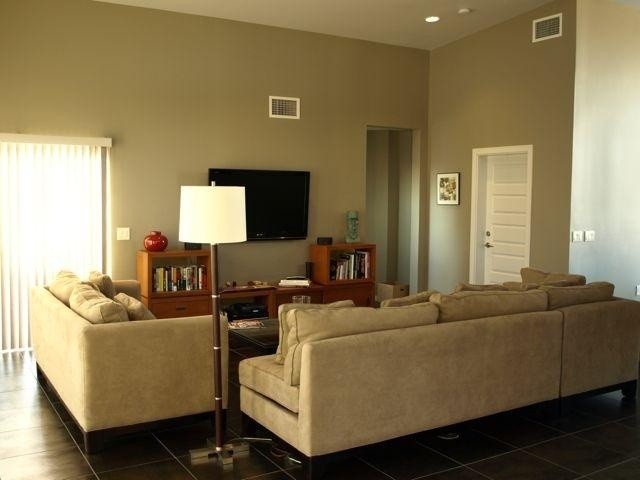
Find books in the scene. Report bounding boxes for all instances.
[328,249,371,281]
[227,319,268,330]
[277,274,313,289]
[152,263,208,293]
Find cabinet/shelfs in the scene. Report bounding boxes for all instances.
[136,242,377,373]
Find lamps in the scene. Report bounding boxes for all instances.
[179,181,249,465]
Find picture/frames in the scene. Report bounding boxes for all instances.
[435,172,461,206]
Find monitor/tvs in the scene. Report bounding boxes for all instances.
[207,168,310,241]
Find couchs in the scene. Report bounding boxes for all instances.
[239,297,640,479]
[28,279,228,453]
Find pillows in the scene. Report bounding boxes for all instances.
[452,280,540,292]
[89,270,117,298]
[536,281,615,308]
[279,299,358,309]
[377,289,442,305]
[519,267,588,288]
[48,267,82,305]
[425,288,551,317]
[68,282,129,324]
[113,291,157,320]
[281,300,443,385]
